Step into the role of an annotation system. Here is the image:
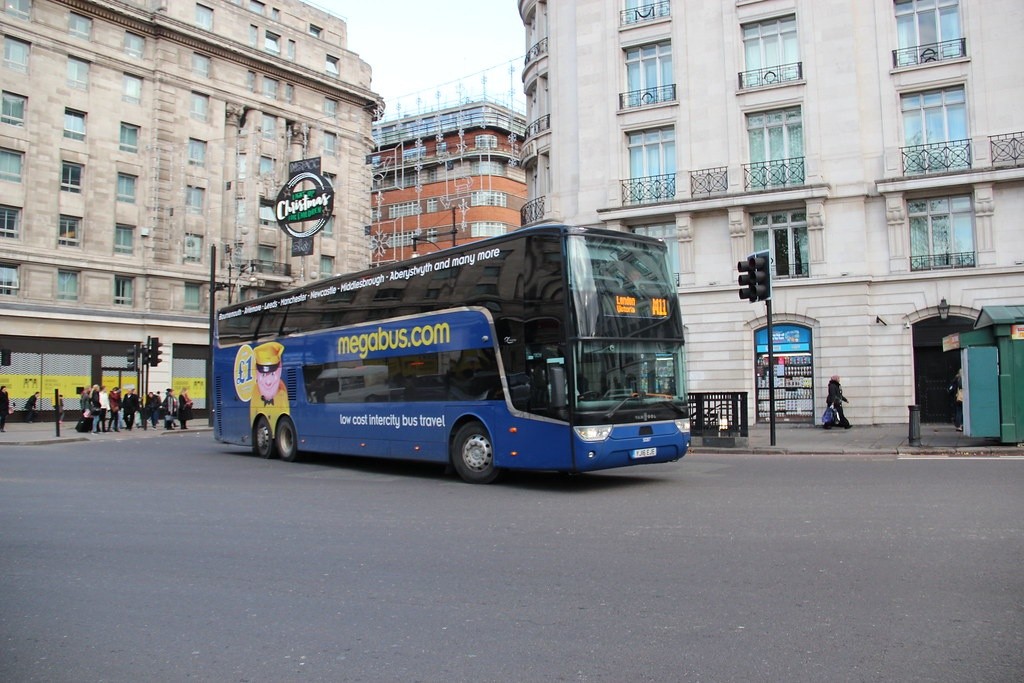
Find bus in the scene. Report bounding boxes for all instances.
[213,223,690,484]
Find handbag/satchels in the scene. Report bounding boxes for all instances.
[91,407,102,415]
[822,407,840,424]
[112,406,119,412]
[9,407,14,415]
[956,387,962,403]
[83,409,92,418]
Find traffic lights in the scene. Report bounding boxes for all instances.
[126,345,136,371]
[144,337,163,366]
[738,250,772,302]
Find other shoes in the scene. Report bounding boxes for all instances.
[844,425,853,429]
[823,425,831,429]
[956,426,963,432]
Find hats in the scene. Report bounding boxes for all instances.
[831,375,840,380]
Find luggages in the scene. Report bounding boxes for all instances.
[75,417,94,432]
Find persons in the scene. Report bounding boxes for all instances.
[0,386,9,432]
[96,386,110,432]
[955,369,963,432]
[823,375,853,429]
[24,392,39,423]
[178,388,193,429]
[74,384,102,433]
[147,388,179,430]
[59,395,63,424]
[122,389,139,431]
[107,387,124,432]
